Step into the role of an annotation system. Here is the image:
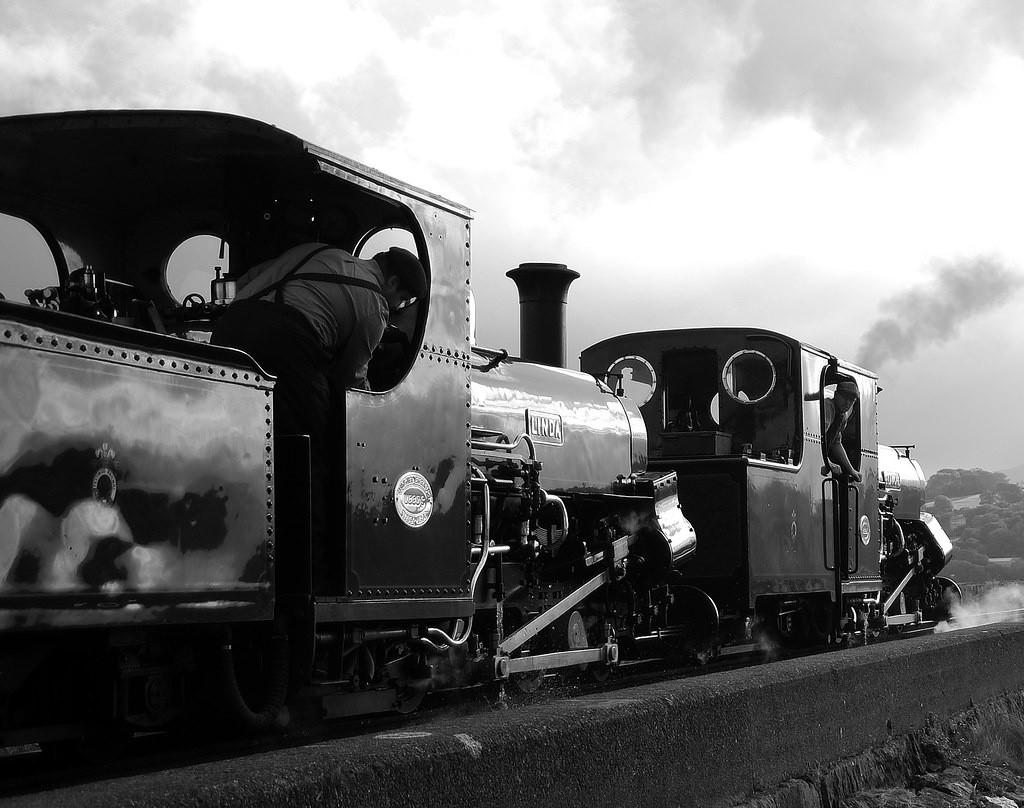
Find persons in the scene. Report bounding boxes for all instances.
[209,242,427,595]
[825,382,863,481]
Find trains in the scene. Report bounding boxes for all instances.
[0,109,966,766]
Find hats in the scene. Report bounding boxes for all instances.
[389,246,428,301]
[837,381,860,399]
